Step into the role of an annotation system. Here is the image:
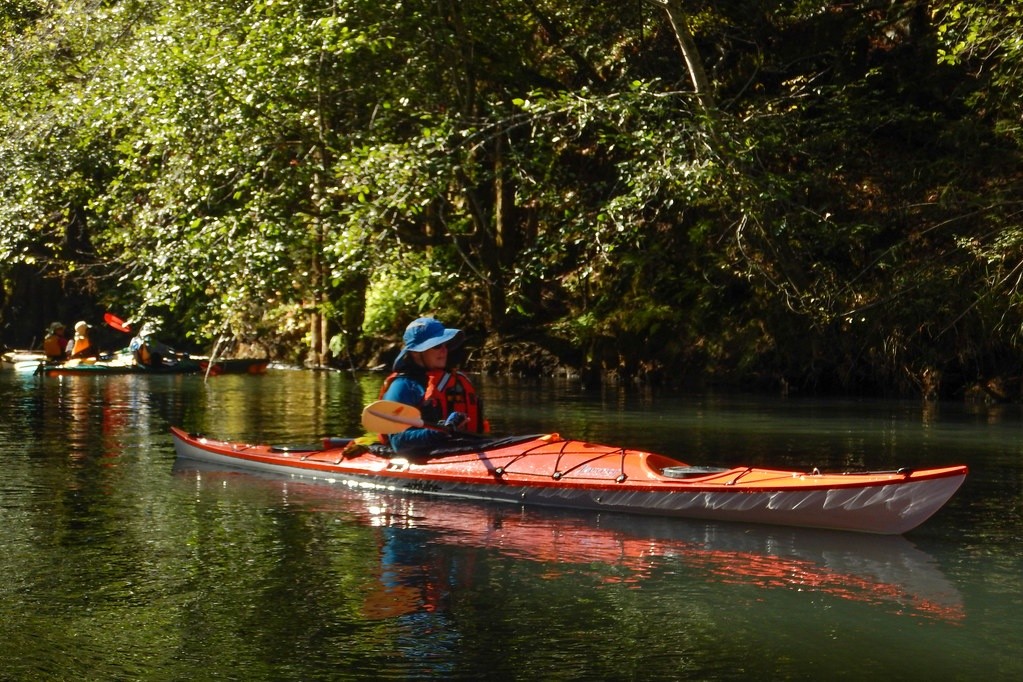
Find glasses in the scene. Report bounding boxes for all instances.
[434,344,443,349]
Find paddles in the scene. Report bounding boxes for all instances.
[105,312,218,374]
[361,400,463,438]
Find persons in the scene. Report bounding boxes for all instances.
[75,320,101,361]
[44,322,74,361]
[381,316,484,456]
[132,321,176,366]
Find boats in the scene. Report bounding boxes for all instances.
[0,348,49,363]
[11,356,272,378]
[166,418,970,537]
[170,454,970,631]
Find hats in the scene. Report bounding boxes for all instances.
[46,322,65,335]
[392,317,463,372]
[74,321,92,335]
[140,322,161,337]
